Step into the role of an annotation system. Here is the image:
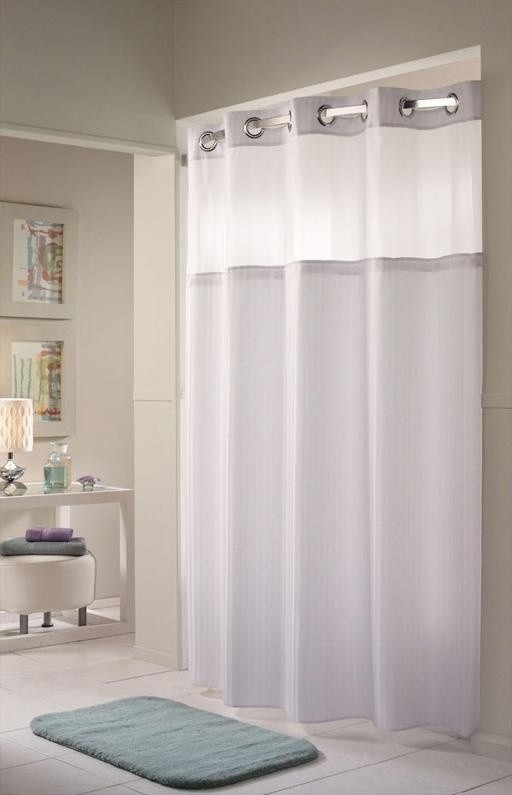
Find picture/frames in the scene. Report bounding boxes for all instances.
[1,322,79,439]
[0,199,80,322]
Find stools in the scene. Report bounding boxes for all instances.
[0,549,97,635]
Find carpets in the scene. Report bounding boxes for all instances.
[31,695,321,791]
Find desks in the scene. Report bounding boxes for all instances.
[0,479,134,654]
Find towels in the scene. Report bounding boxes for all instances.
[0,537,87,557]
[25,527,74,542]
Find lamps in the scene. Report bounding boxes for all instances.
[0,398,35,484]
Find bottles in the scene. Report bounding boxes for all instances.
[50,442,72,488]
[44,453,68,492]
